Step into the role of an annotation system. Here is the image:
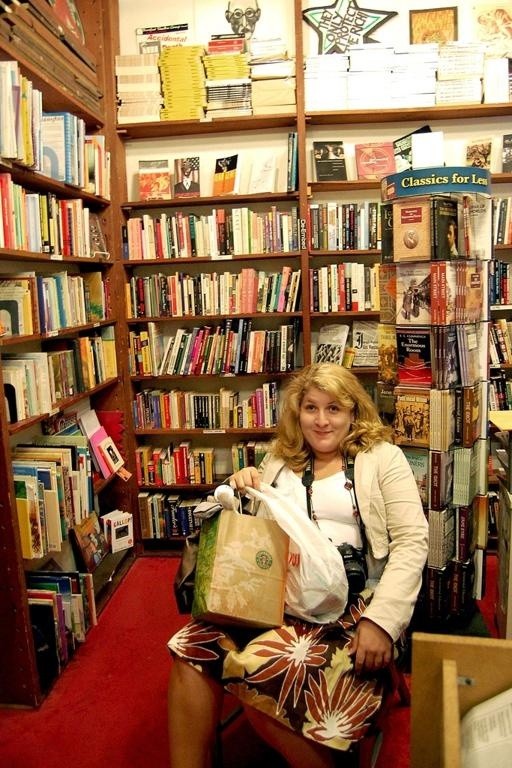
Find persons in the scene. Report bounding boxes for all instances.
[167,361,429,768]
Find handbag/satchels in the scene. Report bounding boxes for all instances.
[174,509,290,628]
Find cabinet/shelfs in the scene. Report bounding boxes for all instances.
[120,106,512,557]
[2,47,142,709]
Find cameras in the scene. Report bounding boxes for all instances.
[337,542,369,593]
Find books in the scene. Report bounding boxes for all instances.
[0,61,126,683]
[128,132,302,540]
[114,32,297,124]
[304,42,512,112]
[309,125,512,635]
